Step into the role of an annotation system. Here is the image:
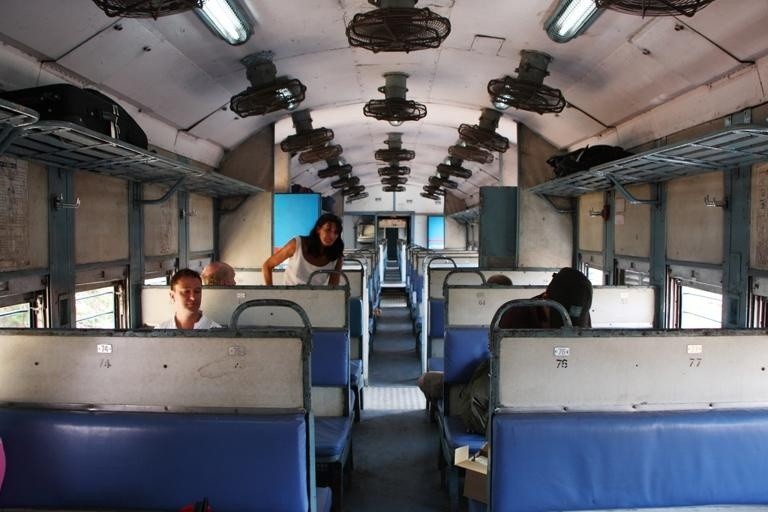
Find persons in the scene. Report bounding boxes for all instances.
[154,269,222,329]
[418,275,513,403]
[200,262,236,286]
[461,297,552,435]
[529,267,592,330]
[263,214,344,287]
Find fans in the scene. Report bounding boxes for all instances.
[488,50,567,116]
[229,57,308,118]
[363,73,427,126]
[421,109,510,201]
[595,0,714,18]
[279,110,370,201]
[92,0,200,20]
[374,139,416,192]
[345,1,453,53]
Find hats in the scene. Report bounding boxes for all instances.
[549,265,593,329]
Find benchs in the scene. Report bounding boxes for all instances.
[1,239,386,511]
[396,235,768,512]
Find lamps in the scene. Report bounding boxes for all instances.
[546,2,599,43]
[196,0,253,46]
